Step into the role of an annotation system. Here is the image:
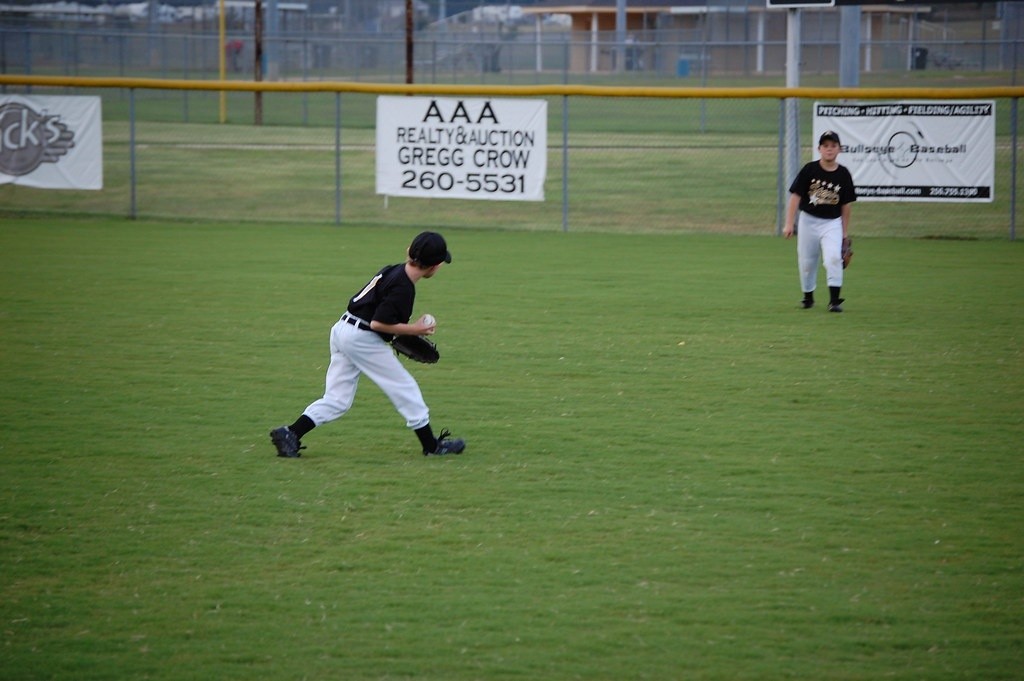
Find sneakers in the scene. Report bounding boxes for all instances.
[270,428,301,457]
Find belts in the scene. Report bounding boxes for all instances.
[342,314,374,332]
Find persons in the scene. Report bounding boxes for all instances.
[270,231,466,458]
[782,130,857,313]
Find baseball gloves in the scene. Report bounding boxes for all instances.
[842,237,853,269]
[392,333,441,364]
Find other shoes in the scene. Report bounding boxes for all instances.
[423,438,465,456]
[829,304,841,312]
[802,302,813,308]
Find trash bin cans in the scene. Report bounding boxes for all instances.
[911,47,927,71]
[483,44,502,73]
[312,41,331,68]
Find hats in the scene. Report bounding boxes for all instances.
[819,132,839,143]
[409,231,451,264]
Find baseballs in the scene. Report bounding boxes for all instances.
[424,314,436,326]
